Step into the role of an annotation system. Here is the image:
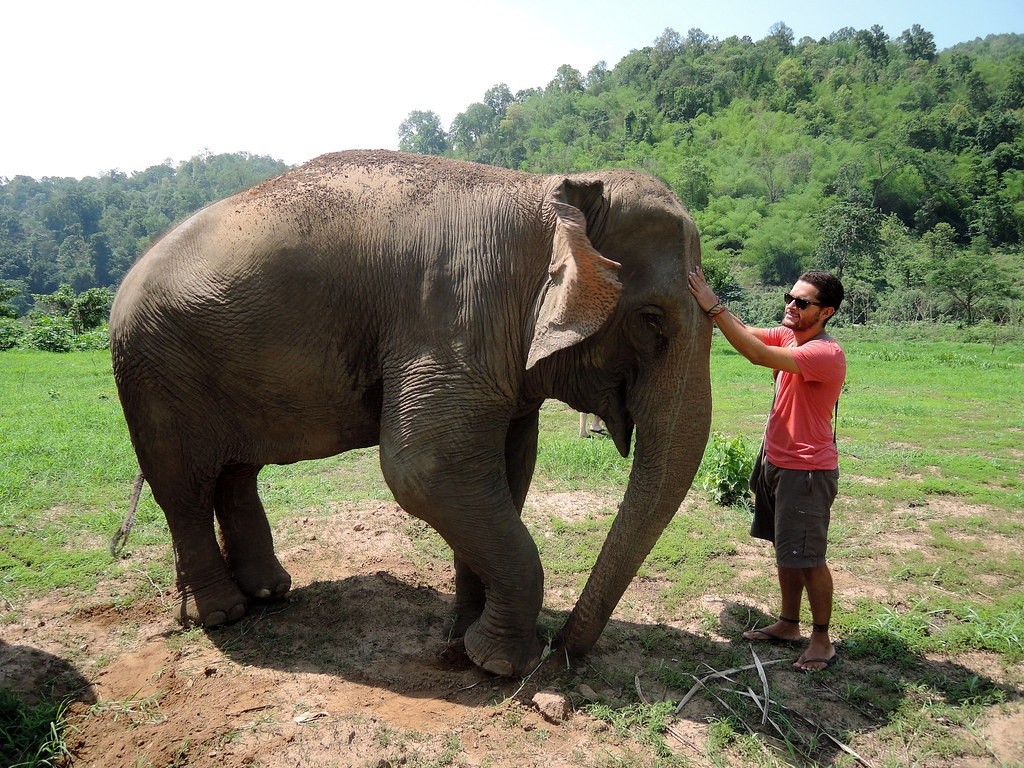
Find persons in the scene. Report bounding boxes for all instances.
[687,264,846,674]
[579,411,609,439]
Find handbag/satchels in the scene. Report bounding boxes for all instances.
[749,424,768,495]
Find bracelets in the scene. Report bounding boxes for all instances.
[706,303,726,319]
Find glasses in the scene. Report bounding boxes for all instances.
[784,293,821,310]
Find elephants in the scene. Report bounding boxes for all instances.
[108,148,713,687]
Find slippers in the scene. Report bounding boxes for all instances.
[742,629,803,645]
[792,654,839,675]
[579,435,595,439]
[590,429,610,436]
